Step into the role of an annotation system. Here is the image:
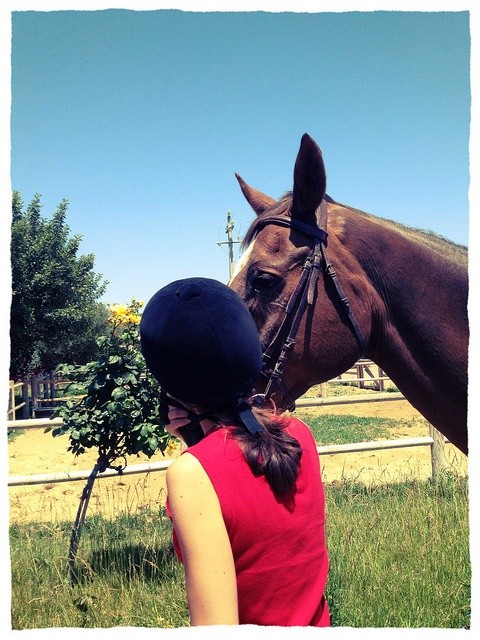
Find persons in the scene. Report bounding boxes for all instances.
[139,277,331,629]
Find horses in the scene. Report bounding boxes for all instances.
[178,132,469,459]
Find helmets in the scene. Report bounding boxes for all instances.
[139,277,263,405]
